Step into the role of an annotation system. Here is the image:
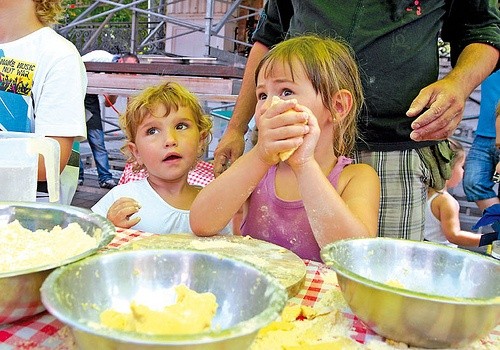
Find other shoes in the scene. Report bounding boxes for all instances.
[78,179,83,186]
[100,179,118,189]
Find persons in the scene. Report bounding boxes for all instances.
[190,33,381,263]
[67,49,140,188]
[424,138,484,249]
[462,69,500,212]
[89,80,233,237]
[213,0,500,240]
[0,0,89,206]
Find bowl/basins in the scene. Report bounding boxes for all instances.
[320,236,500,350]
[0,201,115,324]
[40,249,289,350]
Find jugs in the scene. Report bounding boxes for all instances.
[0,131,60,203]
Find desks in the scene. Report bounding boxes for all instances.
[0,227,500,350]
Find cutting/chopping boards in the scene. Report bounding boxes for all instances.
[125,234,307,300]
